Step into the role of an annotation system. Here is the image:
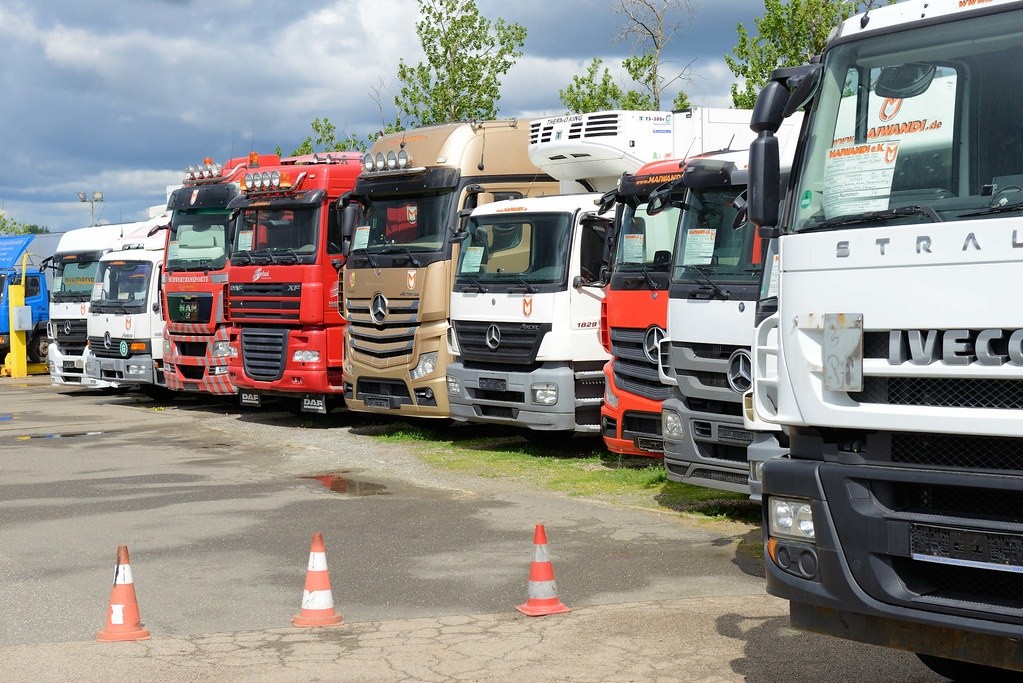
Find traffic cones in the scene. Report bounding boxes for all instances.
[95,546,152,641]
[514,524,572,617]
[290,532,344,626]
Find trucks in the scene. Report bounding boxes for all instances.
[741,0,1023,683]
[0,72,957,494]
